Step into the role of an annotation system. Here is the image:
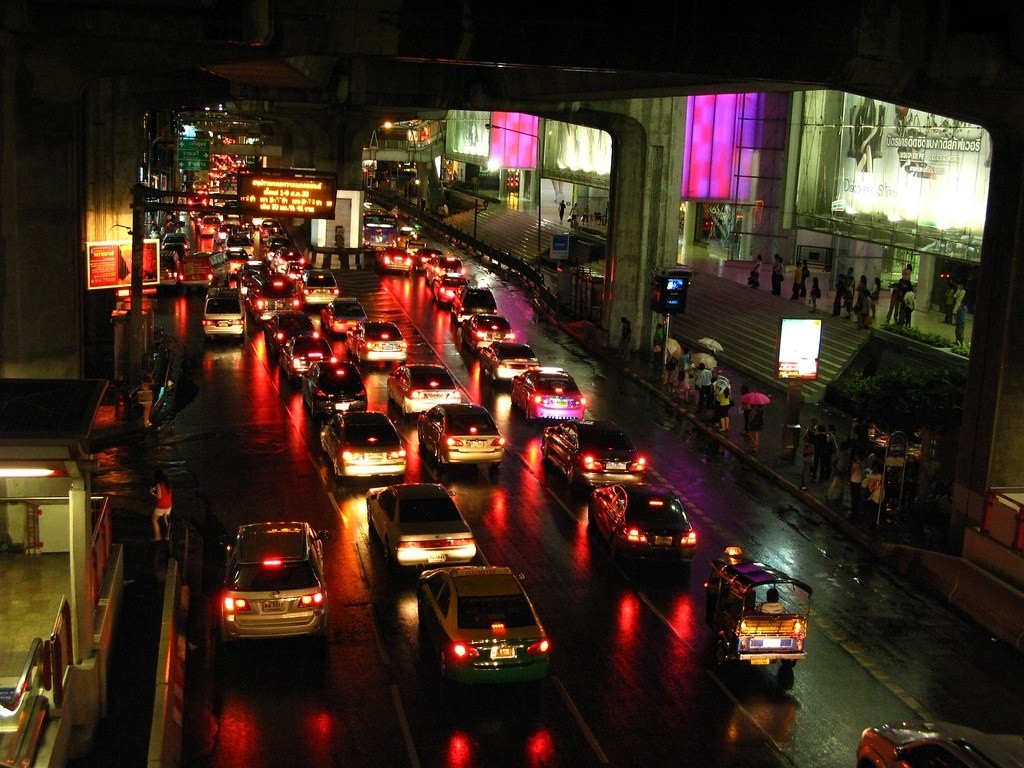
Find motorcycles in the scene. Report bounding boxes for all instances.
[704,556,813,674]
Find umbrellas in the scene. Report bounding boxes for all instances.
[740,393,770,405]
[699,338,724,352]
[693,353,717,368]
[667,338,683,360]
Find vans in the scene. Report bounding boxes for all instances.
[204,285,250,346]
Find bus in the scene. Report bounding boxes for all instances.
[361,210,401,253]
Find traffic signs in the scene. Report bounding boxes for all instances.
[176,138,212,171]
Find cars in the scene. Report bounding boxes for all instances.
[320,299,370,336]
[264,310,317,356]
[462,313,515,354]
[510,364,587,423]
[853,720,1002,768]
[217,521,331,651]
[365,482,479,571]
[157,196,340,324]
[540,418,649,496]
[386,362,463,418]
[302,360,368,419]
[589,484,698,563]
[317,410,408,484]
[417,563,559,691]
[453,285,498,323]
[420,401,506,474]
[478,340,541,386]
[276,336,335,380]
[378,245,414,274]
[346,318,408,367]
[434,274,468,304]
[400,220,465,285]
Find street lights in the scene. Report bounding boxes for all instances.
[483,122,544,270]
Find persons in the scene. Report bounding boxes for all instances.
[900,264,912,282]
[654,323,663,362]
[560,200,566,221]
[810,277,818,312]
[791,260,808,300]
[770,254,783,296]
[570,203,580,221]
[757,589,783,615]
[615,317,632,363]
[945,283,968,346]
[668,347,731,432]
[801,419,887,519]
[583,204,590,226]
[149,468,173,542]
[832,268,881,330]
[142,375,155,427]
[740,384,764,451]
[886,279,915,328]
[750,255,763,288]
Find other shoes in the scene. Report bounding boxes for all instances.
[719,428,725,431]
[844,315,851,320]
[742,431,748,435]
[725,428,728,431]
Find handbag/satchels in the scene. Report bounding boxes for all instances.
[864,317,872,326]
[805,269,810,278]
[729,399,735,408]
[816,287,822,300]
